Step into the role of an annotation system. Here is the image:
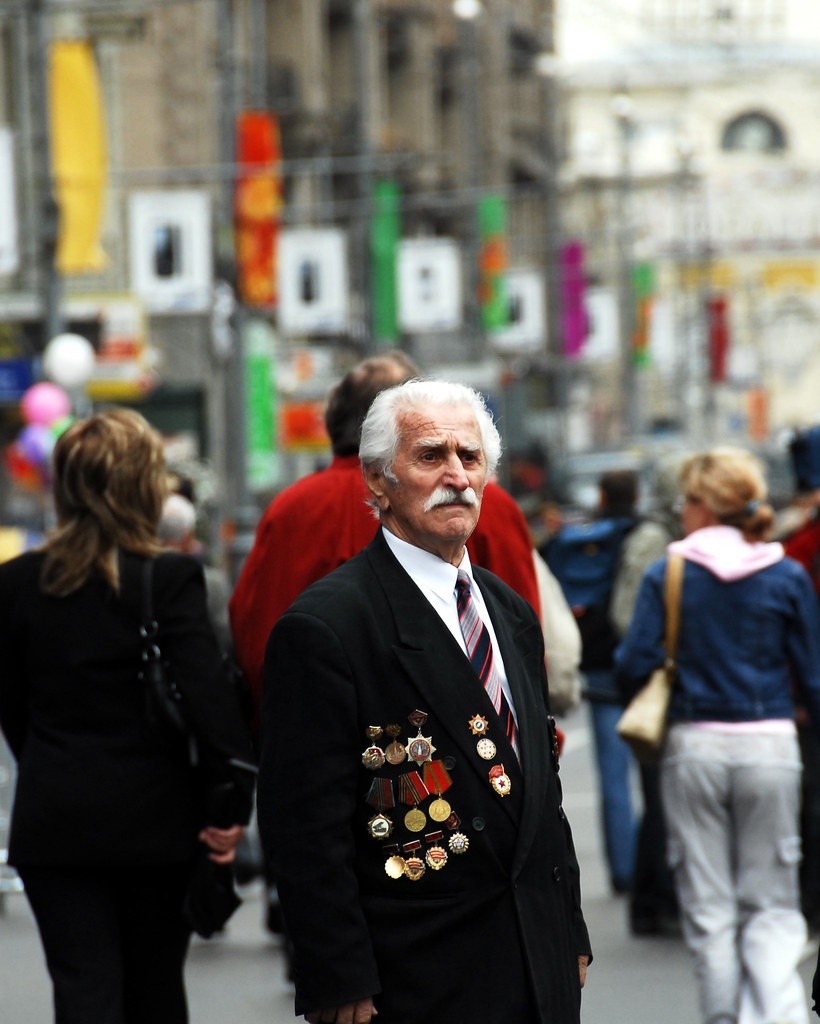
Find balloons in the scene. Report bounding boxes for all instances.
[41,332,96,390]
[4,385,81,492]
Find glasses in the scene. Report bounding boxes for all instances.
[671,495,701,515]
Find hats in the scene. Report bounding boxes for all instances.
[788,426,820,488]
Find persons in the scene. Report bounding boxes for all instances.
[611,449,820,1024]
[0,410,255,1024]
[228,350,541,679]
[260,385,595,1024]
[530,553,583,714]
[537,421,820,1011]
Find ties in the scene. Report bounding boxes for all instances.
[455,569,524,776]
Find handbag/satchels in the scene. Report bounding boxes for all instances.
[616,541,685,758]
[183,767,242,939]
